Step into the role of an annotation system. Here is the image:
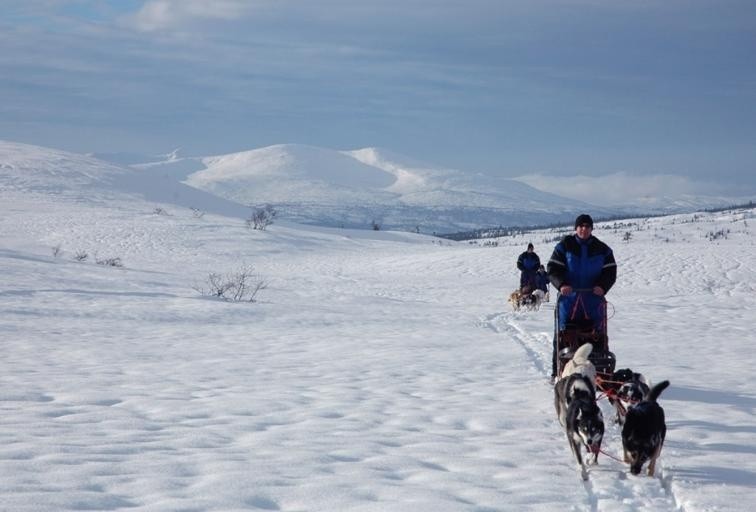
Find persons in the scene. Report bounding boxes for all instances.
[547,212,618,378]
[517,242,551,293]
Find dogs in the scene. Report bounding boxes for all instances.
[507,288,547,312]
[552,340,671,479]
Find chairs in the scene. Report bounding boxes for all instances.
[560,317,615,375]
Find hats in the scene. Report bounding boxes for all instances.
[574,214,594,231]
